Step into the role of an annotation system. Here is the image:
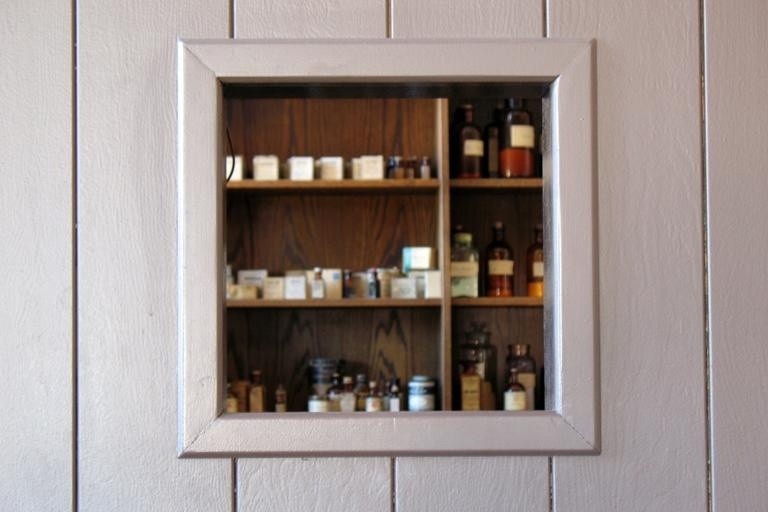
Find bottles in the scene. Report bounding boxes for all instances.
[310,267,326,300]
[223,357,437,413]
[450,232,480,298]
[525,223,546,297]
[453,320,537,412]
[387,155,432,179]
[482,220,515,296]
[342,268,355,297]
[450,95,537,179]
[368,267,381,297]
